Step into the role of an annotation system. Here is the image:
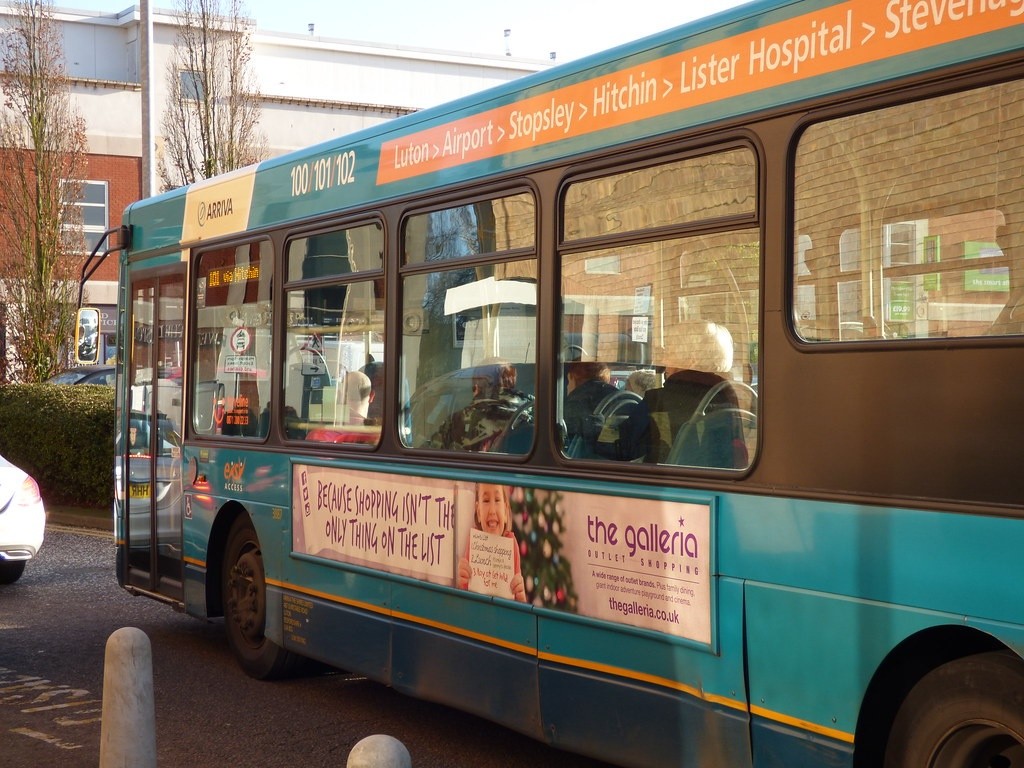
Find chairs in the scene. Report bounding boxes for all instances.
[489,378,759,470]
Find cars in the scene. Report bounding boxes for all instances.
[39,364,116,388]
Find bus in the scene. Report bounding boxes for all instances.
[74,0,1024,768]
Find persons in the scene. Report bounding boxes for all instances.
[457,482,527,604]
[216,318,759,469]
[157,360,172,378]
